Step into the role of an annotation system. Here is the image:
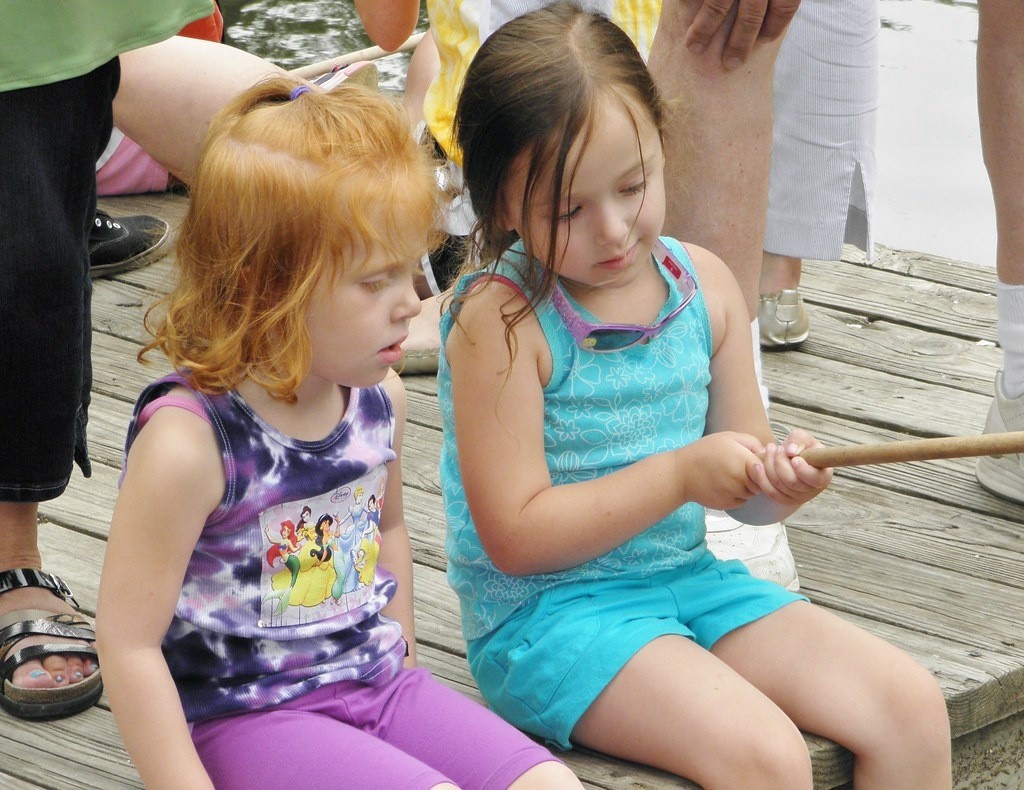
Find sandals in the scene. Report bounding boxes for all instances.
[0,568,103,721]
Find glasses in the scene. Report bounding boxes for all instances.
[541,236,697,353]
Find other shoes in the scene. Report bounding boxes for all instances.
[389,286,459,374]
[976,372,1024,507]
[757,288,810,351]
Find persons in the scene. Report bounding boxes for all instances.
[87,0,1024,515]
[97,75,582,790]
[0,0,212,722]
[438,4,952,790]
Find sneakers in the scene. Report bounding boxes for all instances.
[87,208,172,279]
